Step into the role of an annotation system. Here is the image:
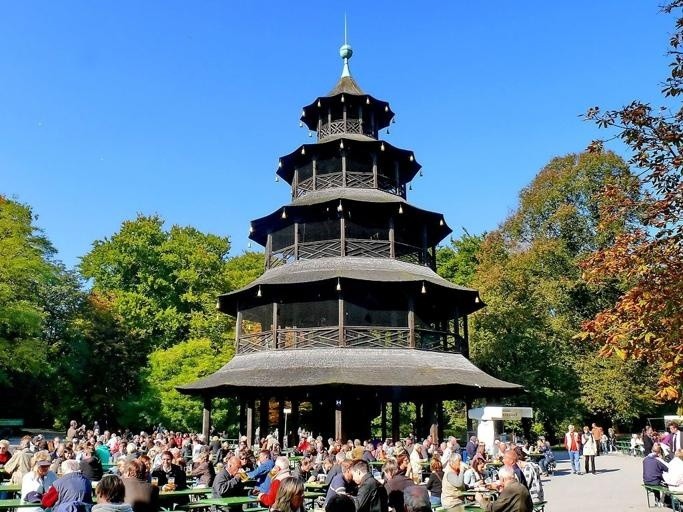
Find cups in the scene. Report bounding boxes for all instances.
[151,477,158,486]
[483,493,490,502]
[168,478,174,492]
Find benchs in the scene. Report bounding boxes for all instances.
[615,436,645,457]
[641,483,683,512]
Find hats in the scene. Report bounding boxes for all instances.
[37,460,52,466]
[652,443,660,451]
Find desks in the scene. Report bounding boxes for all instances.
[0,437,552,512]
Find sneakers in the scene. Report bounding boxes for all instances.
[572,471,583,475]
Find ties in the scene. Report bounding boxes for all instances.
[674,435,676,452]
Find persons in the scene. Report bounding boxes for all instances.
[631,420,682,511]
[1,420,554,512]
[564,422,617,476]
